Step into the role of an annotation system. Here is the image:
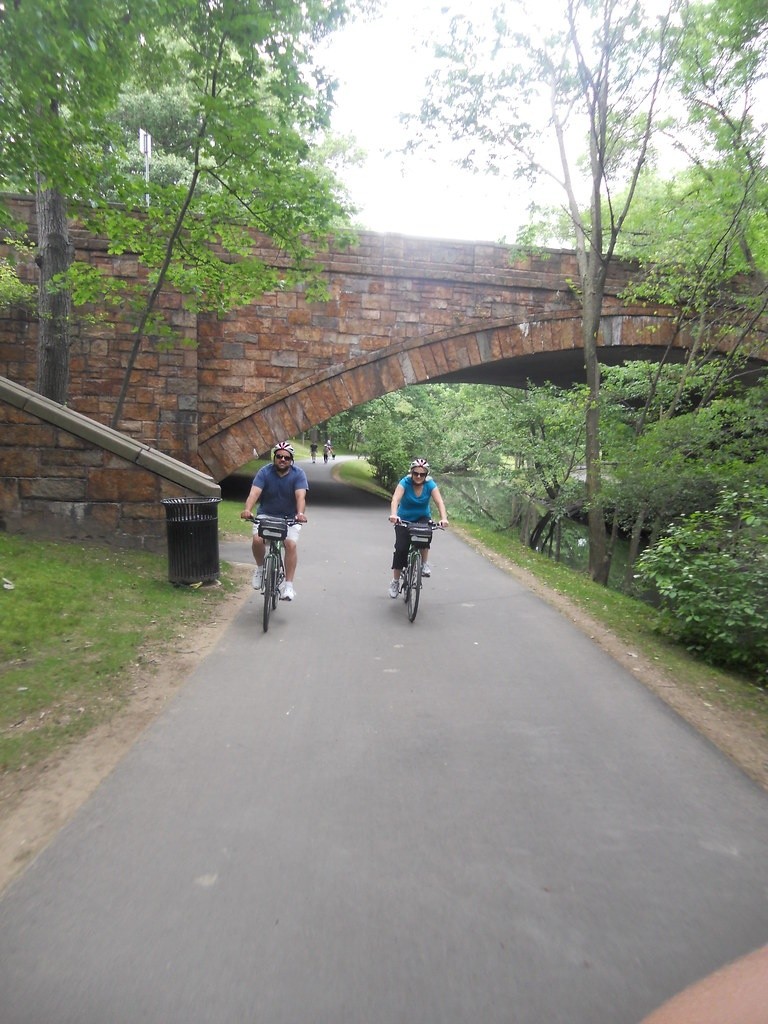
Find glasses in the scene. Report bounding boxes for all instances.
[413,470,427,477]
[275,454,292,461]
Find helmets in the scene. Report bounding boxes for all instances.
[410,459,430,474]
[273,442,295,461]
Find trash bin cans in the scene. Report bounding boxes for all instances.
[160,497,223,585]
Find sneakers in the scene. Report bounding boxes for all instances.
[421,562,431,577]
[251,568,263,589]
[281,586,294,600]
[389,581,400,598]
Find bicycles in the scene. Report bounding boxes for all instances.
[388,515,446,623]
[240,512,308,633]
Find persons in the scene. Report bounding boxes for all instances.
[389,458,449,599]
[310,440,318,463]
[324,439,332,464]
[241,441,310,602]
